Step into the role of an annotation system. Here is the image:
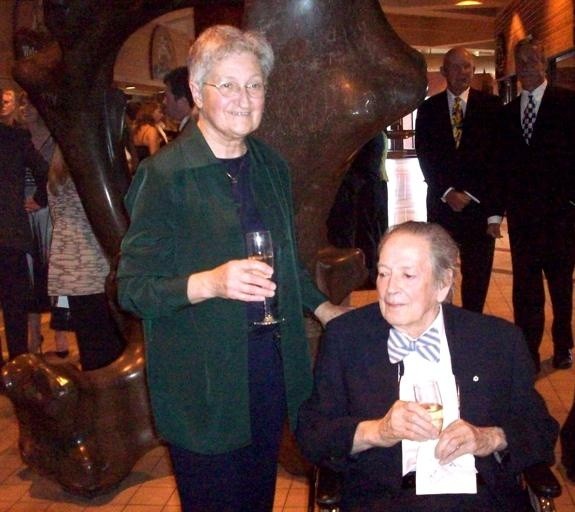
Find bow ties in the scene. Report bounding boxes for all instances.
[387,327,441,365]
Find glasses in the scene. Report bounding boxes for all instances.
[203,80,268,99]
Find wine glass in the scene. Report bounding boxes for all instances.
[413,380,456,468]
[243,231,285,326]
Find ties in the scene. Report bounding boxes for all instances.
[451,96,464,151]
[522,95,537,146]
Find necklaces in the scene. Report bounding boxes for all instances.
[215,147,248,185]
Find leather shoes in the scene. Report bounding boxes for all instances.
[552,344,572,369]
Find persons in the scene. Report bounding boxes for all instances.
[112,21,317,511]
[1,63,200,400]
[485,36,575,372]
[295,219,560,511]
[414,44,506,316]
